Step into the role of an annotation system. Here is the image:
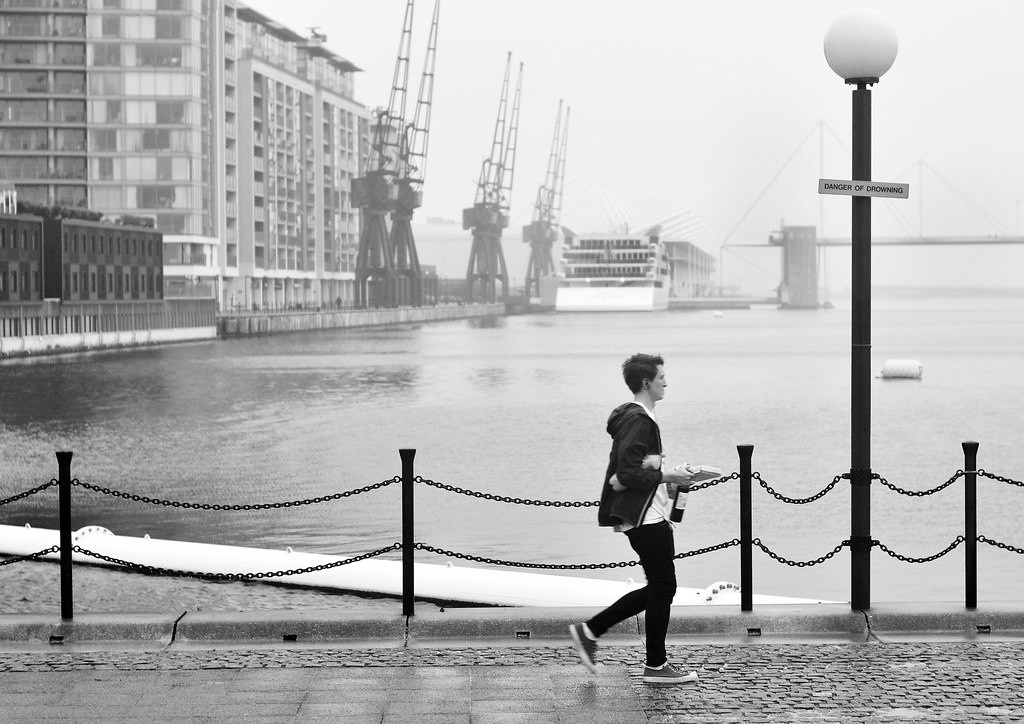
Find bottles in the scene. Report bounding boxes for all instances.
[670,470,693,523]
[609,452,666,493]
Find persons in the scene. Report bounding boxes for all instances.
[568,354,699,683]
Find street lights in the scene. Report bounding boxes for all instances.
[823,11,901,609]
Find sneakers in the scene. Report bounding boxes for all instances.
[569,623,598,674]
[642,661,699,683]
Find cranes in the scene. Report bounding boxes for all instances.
[349,1,572,300]
